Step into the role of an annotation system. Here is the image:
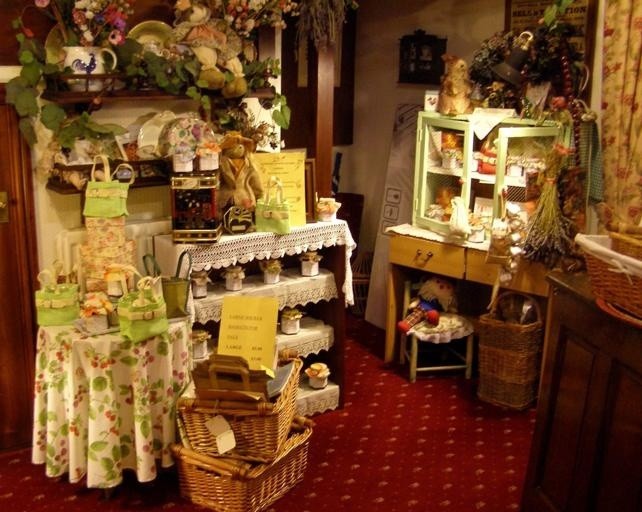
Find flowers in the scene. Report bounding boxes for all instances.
[18,0,135,48]
[215,0,300,39]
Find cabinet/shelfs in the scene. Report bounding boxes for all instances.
[154,217,350,417]
[411,109,562,245]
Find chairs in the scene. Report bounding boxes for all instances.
[400,277,475,383]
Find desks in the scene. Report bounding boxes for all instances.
[518,255,641,511]
[32,316,192,490]
[383,225,562,410]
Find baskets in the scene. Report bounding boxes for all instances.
[175,351,307,465]
[168,416,322,512]
[571,227,642,320]
[477,290,546,409]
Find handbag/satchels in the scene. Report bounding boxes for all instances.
[252,176,292,234]
[83,153,135,217]
[116,264,170,341]
[140,251,193,318]
[35,256,80,328]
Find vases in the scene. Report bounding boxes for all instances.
[62,46,117,91]
[243,40,258,62]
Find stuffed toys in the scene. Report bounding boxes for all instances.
[172,0,253,99]
[397,276,457,331]
[218,132,264,214]
[437,53,474,116]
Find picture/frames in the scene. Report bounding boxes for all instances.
[505,0,599,109]
